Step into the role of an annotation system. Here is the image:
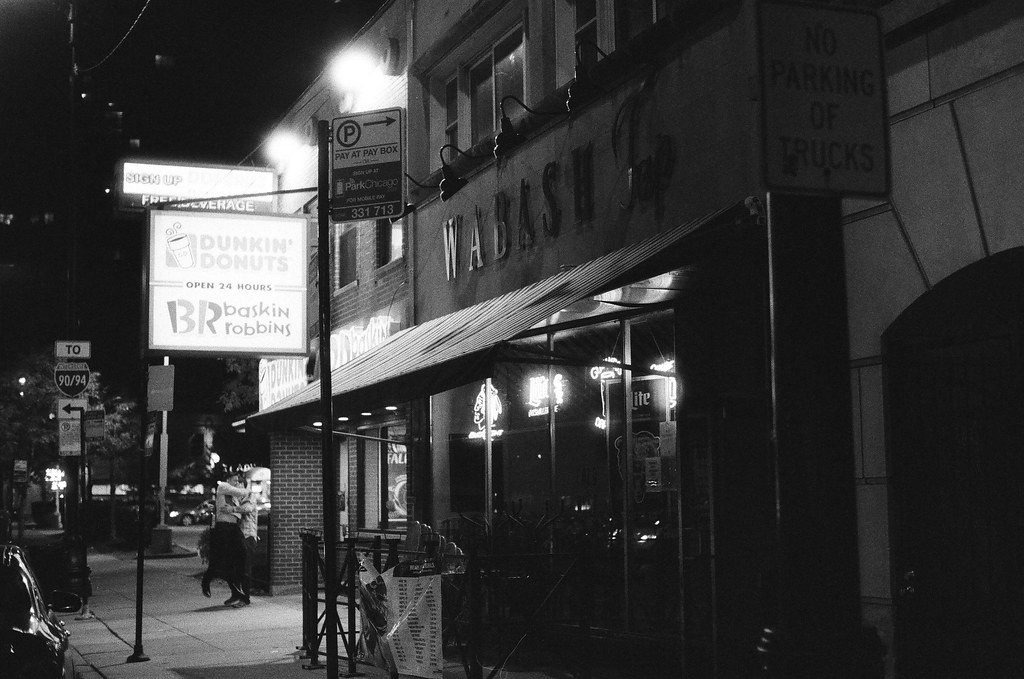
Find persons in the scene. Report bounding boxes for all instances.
[202,473,251,598]
[219,475,260,608]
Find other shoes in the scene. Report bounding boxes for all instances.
[224,596,251,609]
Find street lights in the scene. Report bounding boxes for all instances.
[43,465,67,532]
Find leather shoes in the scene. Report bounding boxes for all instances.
[201,582,211,598]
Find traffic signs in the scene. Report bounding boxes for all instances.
[329,104,407,224]
[82,411,108,444]
[58,398,87,457]
